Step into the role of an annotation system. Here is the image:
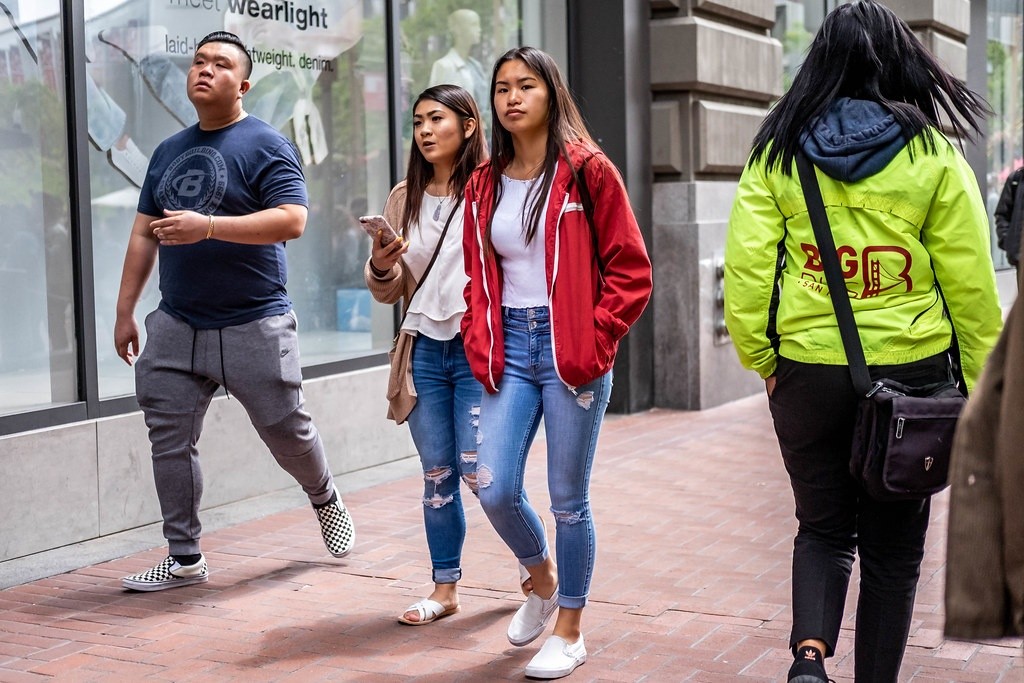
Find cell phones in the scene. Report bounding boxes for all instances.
[359,215,404,251]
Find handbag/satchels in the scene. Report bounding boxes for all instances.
[850,378,968,498]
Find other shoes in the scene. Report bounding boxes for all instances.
[786,645,830,683]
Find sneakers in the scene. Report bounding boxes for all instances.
[524,632,587,680]
[507,581,561,647]
[121,556,208,592]
[313,483,355,559]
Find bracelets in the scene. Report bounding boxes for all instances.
[205,214,217,241]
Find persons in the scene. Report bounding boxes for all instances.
[461,46,652,678]
[363,84,532,627]
[113,32,355,592]
[724,0,1024,683]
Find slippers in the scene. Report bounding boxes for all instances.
[398,599,459,626]
[519,515,548,602]
